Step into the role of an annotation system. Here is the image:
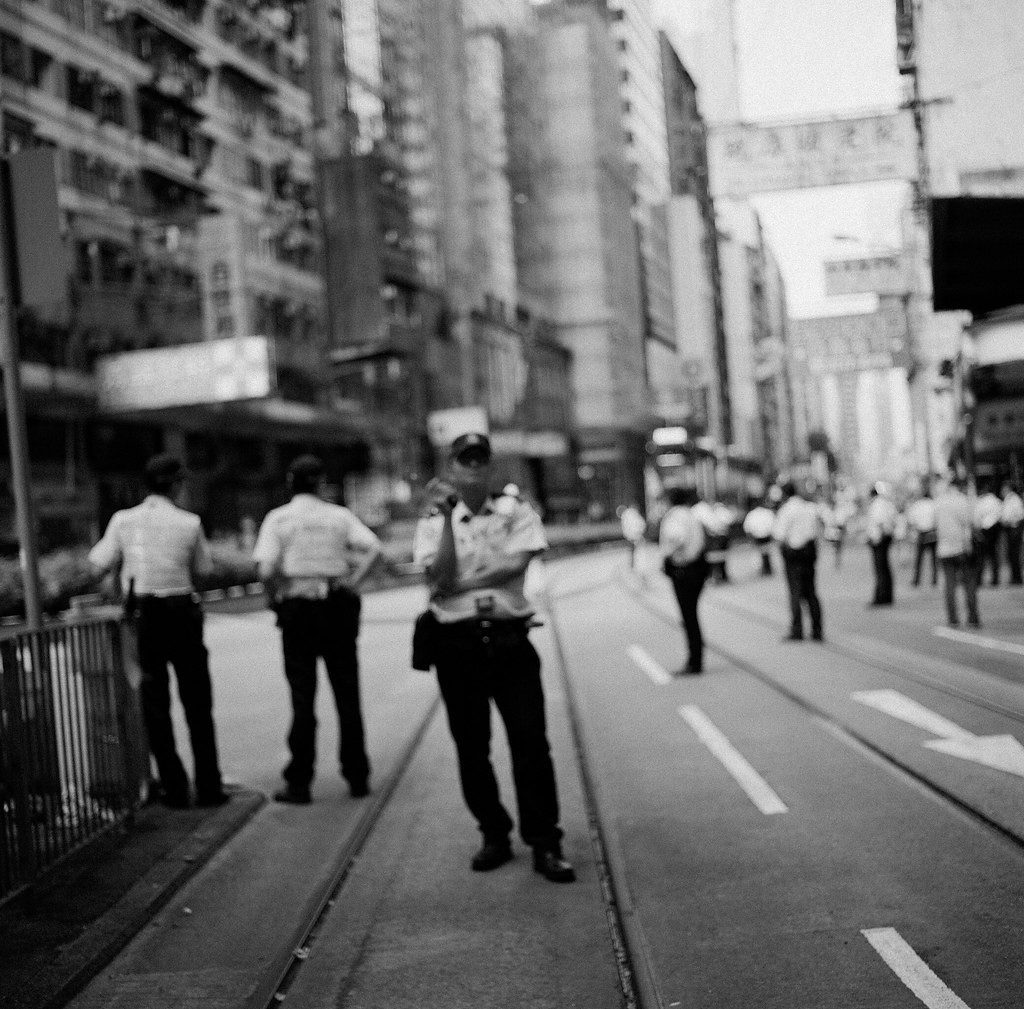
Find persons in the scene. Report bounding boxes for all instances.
[743,501,775,574]
[863,487,899,606]
[251,456,383,803]
[687,489,735,582]
[86,453,229,808]
[771,483,823,642]
[935,475,983,627]
[658,488,709,674]
[909,490,938,587]
[973,482,1024,585]
[409,432,576,882]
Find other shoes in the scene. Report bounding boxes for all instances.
[196,790,229,807]
[274,785,311,803]
[790,635,802,639]
[472,843,514,871]
[159,791,191,809]
[872,600,893,604]
[968,620,979,628]
[813,635,822,640]
[349,780,369,797]
[681,669,701,674]
[533,838,575,882]
[949,620,958,627]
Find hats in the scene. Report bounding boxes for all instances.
[451,433,490,462]
[291,456,323,475]
[140,455,183,481]
